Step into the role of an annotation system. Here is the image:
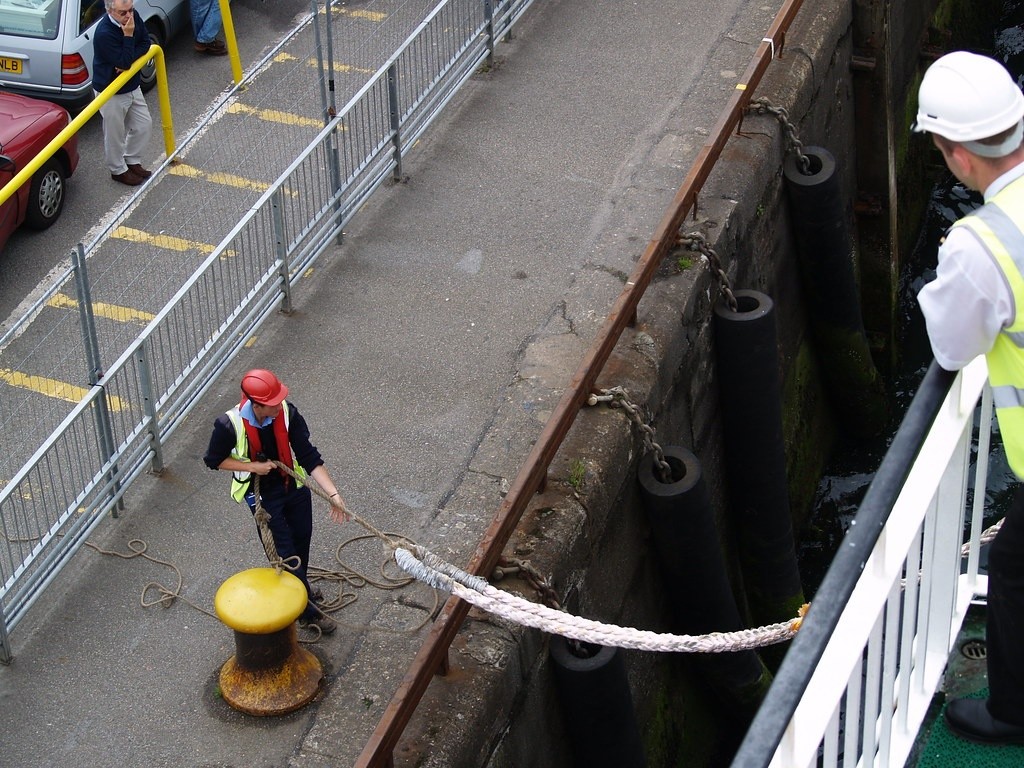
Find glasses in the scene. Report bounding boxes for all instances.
[111,7,133,16]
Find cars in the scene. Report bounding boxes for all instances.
[0,92,78,252]
[0,0,190,101]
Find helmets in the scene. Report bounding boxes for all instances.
[911,49,1023,139]
[241,370,288,407]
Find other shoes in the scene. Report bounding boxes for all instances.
[110,162,150,185]
[194,38,227,57]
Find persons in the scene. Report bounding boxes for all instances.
[915,50,1024,743]
[190,0,232,56]
[92,0,153,186]
[203,370,349,633]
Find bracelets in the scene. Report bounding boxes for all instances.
[330,492,339,498]
